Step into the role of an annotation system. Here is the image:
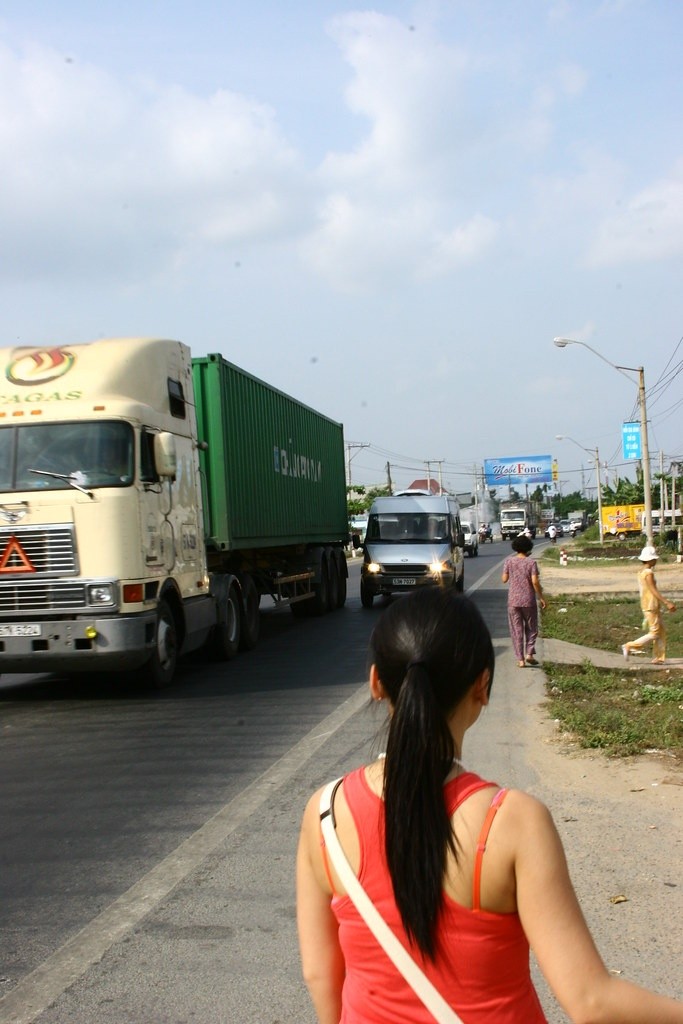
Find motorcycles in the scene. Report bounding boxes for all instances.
[569,528,577,538]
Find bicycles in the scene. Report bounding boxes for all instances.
[551,538,555,545]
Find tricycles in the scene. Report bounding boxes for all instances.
[479,531,493,544]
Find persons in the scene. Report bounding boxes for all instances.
[570,521,576,533]
[478,523,492,541]
[622,545,677,665]
[547,523,557,543]
[502,536,547,668]
[522,528,531,540]
[296,587,683,1024]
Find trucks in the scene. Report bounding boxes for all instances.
[602,504,645,541]
[568,512,587,532]
[497,507,538,541]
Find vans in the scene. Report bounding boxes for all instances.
[352,495,465,607]
[460,522,478,558]
[641,509,683,540]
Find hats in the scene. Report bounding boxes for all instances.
[638,545,659,562]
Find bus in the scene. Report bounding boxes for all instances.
[392,489,432,498]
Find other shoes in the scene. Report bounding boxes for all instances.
[517,656,540,668]
[622,643,631,661]
[651,657,669,665]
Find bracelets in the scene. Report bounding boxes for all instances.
[664,598,670,605]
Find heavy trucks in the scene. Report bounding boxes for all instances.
[1,338,348,692]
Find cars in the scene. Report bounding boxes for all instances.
[559,520,571,532]
[544,523,564,538]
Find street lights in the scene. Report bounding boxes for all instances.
[588,460,608,489]
[555,435,603,545]
[553,337,655,547]
